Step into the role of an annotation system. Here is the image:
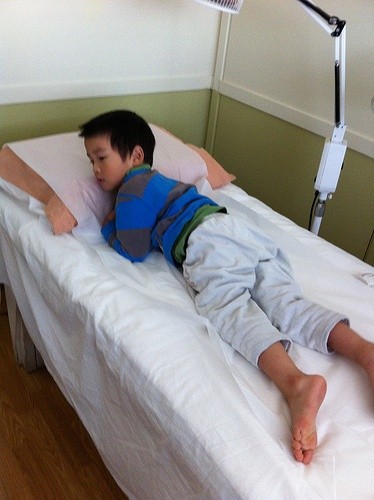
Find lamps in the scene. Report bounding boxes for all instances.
[196,0,348,235]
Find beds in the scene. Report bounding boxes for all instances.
[0,179,374,500]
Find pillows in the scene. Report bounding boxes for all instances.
[0,122,236,236]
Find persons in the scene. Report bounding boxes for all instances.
[75,109,374,465]
